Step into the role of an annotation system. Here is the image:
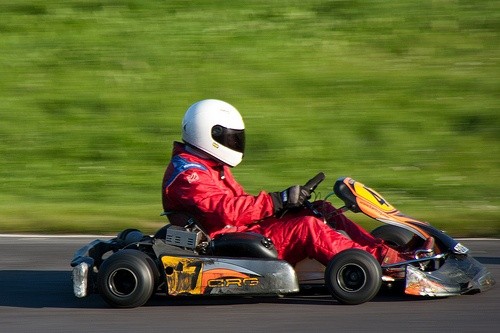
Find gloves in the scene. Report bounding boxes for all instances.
[269,184,313,214]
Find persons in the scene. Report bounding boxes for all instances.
[162,99,435,289]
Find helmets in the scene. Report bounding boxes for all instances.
[182,98,247,166]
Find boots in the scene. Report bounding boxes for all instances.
[382,236,434,280]
[388,234,424,252]
